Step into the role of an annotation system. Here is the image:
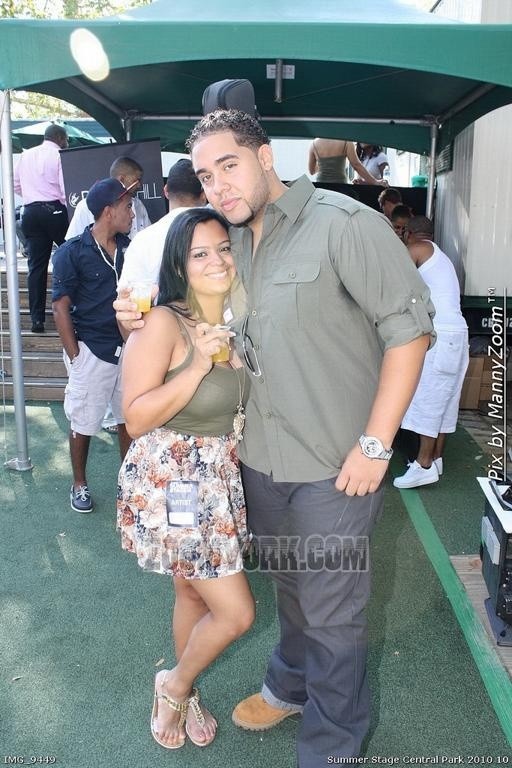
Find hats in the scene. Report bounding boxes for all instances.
[84,176,142,219]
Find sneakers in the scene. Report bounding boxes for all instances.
[433,455,443,477]
[392,459,439,490]
[230,691,297,733]
[69,483,95,513]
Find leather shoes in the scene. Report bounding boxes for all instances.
[31,321,45,333]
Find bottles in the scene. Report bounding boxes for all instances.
[383,166,390,187]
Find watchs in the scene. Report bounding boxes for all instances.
[356,434,394,462]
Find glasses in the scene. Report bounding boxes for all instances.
[231,312,263,380]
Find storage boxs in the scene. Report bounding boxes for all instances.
[458,355,484,410]
[473,353,506,401]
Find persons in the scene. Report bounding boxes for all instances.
[63,157,152,241]
[51,176,133,513]
[116,158,208,312]
[378,187,470,491]
[351,141,390,185]
[308,136,389,188]
[11,124,70,333]
[113,107,437,767]
[115,207,252,748]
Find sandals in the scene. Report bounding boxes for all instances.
[149,668,189,751]
[184,685,219,748]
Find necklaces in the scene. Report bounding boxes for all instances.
[227,358,248,443]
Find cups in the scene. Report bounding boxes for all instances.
[128,280,153,311]
[411,176,428,187]
[208,325,232,363]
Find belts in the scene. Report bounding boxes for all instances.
[29,201,59,207]
